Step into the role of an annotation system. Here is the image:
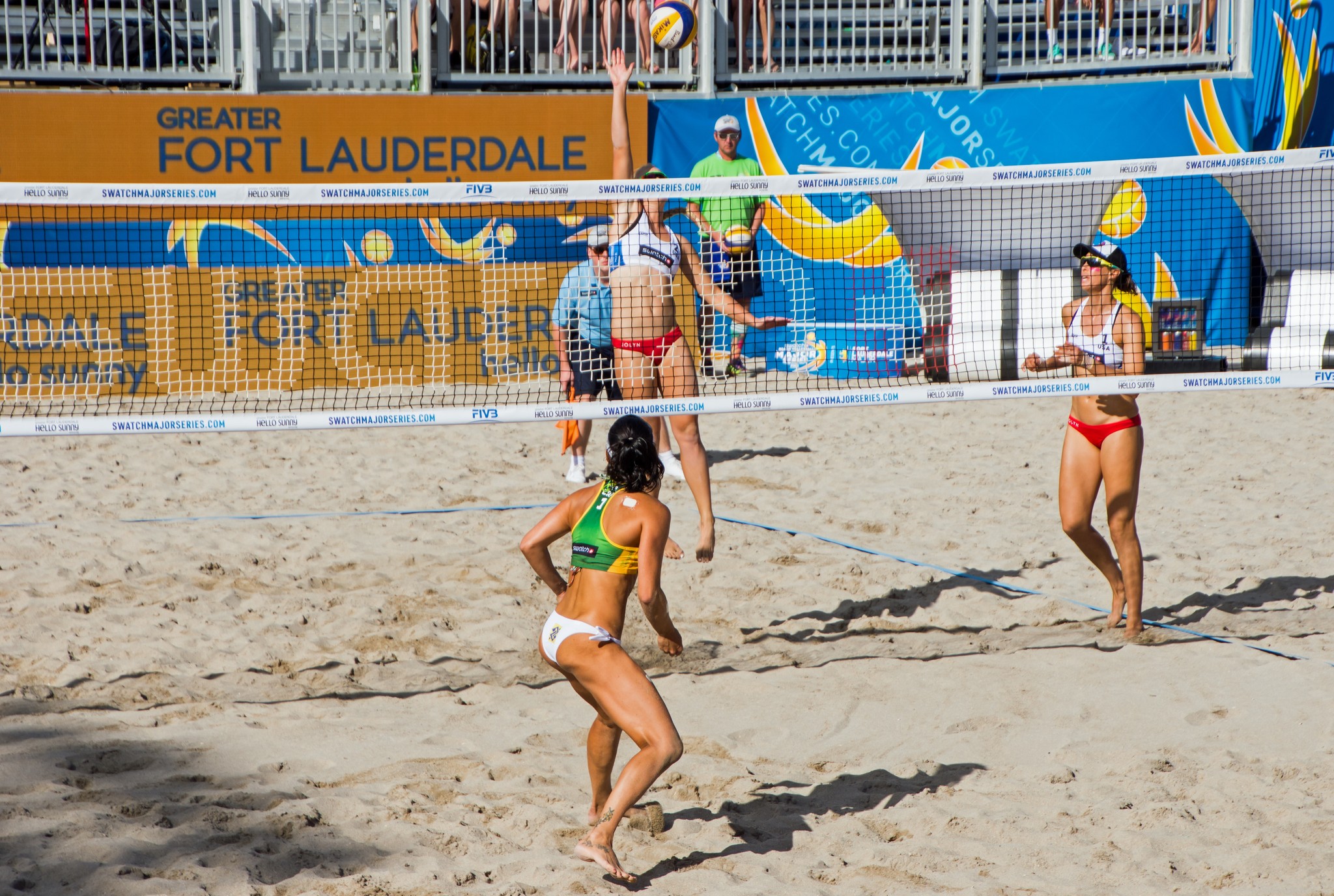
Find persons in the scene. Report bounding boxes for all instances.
[1022,241,1147,644]
[411,0,699,72]
[519,414,683,885]
[1182,0,1216,56]
[602,48,792,564]
[551,224,623,484]
[685,115,770,379]
[1045,0,1117,63]
[727,0,780,73]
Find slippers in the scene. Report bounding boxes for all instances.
[642,61,660,74]
[738,62,754,73]
[761,56,780,72]
[693,62,698,68]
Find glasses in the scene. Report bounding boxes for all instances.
[639,171,668,179]
[1080,256,1124,273]
[588,245,608,255]
[715,130,741,140]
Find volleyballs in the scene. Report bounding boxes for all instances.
[724,224,752,255]
[649,0,698,50]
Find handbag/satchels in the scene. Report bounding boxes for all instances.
[94,18,172,69]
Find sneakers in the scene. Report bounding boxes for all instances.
[1124,36,1146,55]
[1112,36,1134,56]
[565,455,586,483]
[1096,42,1116,61]
[726,359,756,378]
[699,360,717,378]
[1047,44,1063,60]
[658,449,686,480]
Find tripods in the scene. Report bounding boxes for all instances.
[13,0,203,72]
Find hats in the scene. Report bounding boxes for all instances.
[587,226,610,246]
[1073,240,1127,273]
[714,114,741,133]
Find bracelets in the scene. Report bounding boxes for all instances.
[1080,352,1095,369]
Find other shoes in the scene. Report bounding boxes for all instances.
[449,50,462,70]
[480,30,491,50]
[411,49,419,72]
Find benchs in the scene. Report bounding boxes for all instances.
[0,0,1236,89]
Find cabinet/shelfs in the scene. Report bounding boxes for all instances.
[1149,296,1208,360]
